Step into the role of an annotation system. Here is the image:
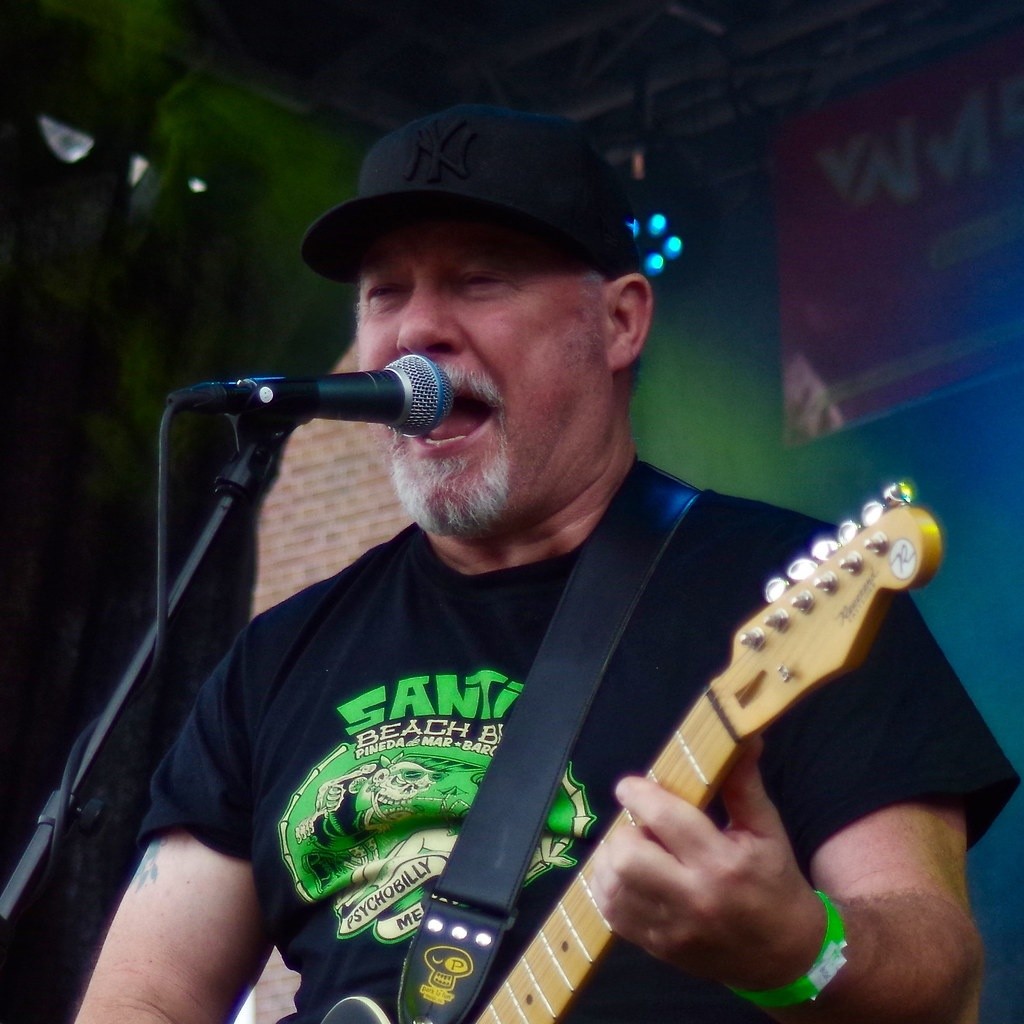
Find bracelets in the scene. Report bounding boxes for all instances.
[723,890,848,1008]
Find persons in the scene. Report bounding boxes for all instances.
[68,102,1024,1023]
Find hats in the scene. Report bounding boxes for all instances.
[300,105,640,284]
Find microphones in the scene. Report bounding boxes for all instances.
[167,356,455,437]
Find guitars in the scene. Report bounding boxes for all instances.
[318,483,950,1024]
